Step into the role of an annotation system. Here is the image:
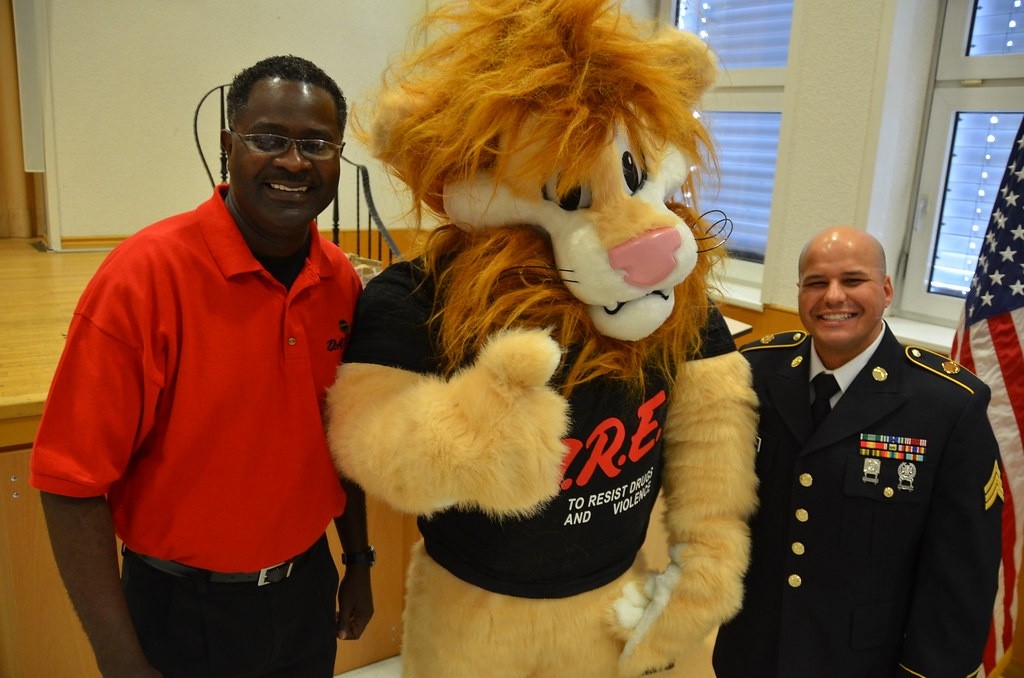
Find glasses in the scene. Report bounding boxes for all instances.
[231,130,344,161]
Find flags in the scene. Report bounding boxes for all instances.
[948,117,1024,678]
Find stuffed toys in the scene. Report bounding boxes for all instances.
[322,0,761,678]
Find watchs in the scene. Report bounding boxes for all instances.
[341,546,376,566]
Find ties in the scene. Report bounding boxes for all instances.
[811,372,841,432]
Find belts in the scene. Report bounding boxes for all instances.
[140,539,334,588]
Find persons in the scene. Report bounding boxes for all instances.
[27,56,375,678]
[712,227,1004,678]
